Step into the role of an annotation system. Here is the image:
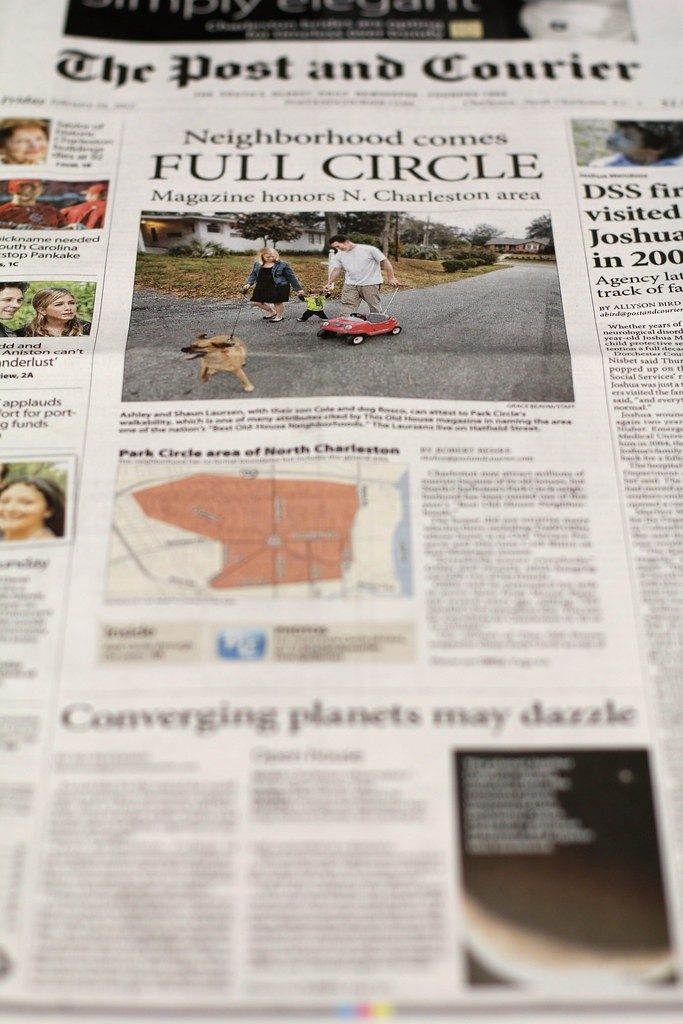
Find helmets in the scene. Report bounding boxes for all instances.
[8,179,52,195]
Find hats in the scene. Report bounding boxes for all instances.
[80,184,108,195]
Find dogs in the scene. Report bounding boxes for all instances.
[182,332,256,393]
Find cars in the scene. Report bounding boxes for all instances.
[317,313,402,345]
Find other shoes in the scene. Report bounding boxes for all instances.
[263,313,277,320]
[296,318,304,322]
[269,317,284,323]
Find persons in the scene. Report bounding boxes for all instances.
[243,235,399,324]
[0,119,48,165]
[0,282,91,337]
[588,120,683,167]
[0,179,107,230]
[0,477,66,542]
